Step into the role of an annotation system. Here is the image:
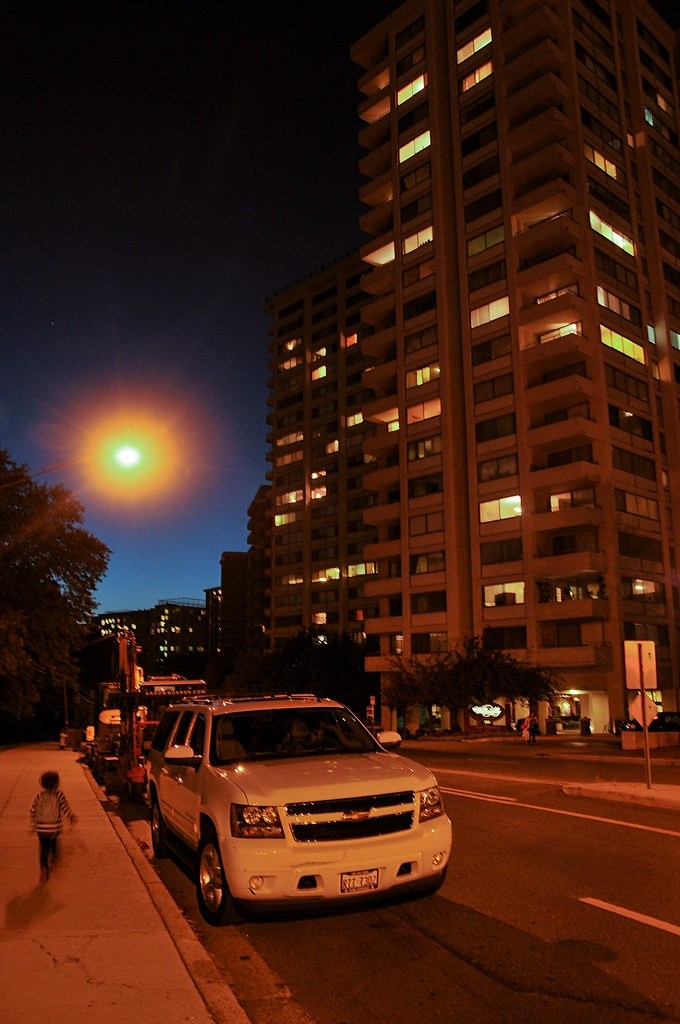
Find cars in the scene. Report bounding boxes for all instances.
[624,711,680,732]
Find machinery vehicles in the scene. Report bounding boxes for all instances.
[68,630,208,802]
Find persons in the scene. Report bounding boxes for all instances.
[525,711,538,744]
[276,720,350,754]
[30,769,77,885]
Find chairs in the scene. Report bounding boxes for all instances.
[285,717,312,751]
[220,719,246,759]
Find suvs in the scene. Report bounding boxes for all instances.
[143,690,455,927]
